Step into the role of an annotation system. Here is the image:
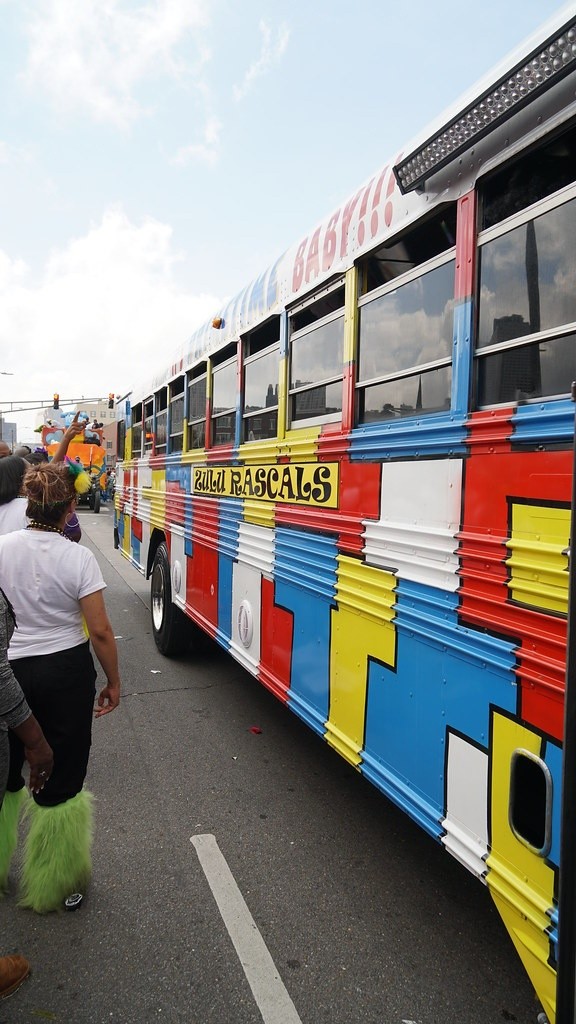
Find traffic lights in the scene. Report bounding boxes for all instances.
[53,394,59,409]
[109,392,115,409]
[146,431,152,440]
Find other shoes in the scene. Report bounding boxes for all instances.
[0,955,31,1000]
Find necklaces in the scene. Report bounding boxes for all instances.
[16,495,28,498]
[27,522,68,539]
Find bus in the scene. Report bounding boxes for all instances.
[109,2,575,1024]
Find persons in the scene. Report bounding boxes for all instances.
[0,410,120,1001]
